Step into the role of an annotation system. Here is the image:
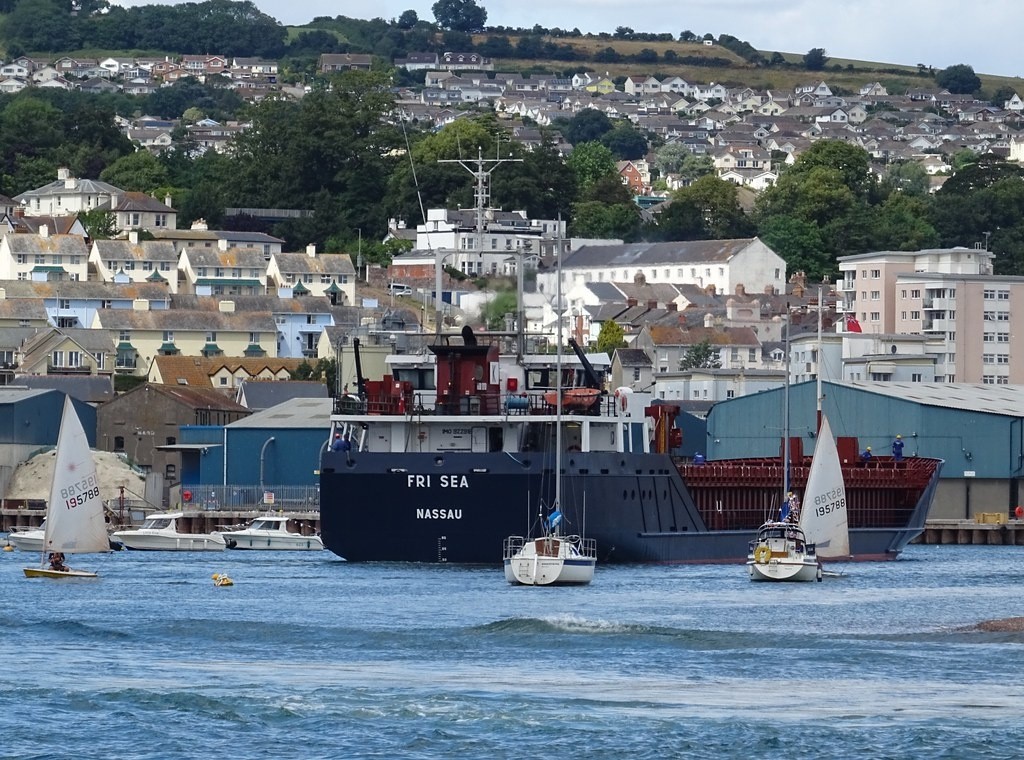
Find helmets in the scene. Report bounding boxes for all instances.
[896,435,902,438]
[335,434,340,438]
[867,447,872,450]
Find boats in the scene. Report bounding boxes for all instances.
[3,514,123,554]
[214,509,324,551]
[113,509,237,551]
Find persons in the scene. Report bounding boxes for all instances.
[49,552,65,570]
[858,447,873,461]
[892,434,904,461]
[328,434,351,465]
[693,450,706,463]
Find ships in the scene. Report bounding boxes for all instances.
[318,244,947,568]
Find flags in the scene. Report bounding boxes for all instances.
[847,314,862,333]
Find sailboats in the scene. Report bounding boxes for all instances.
[501,211,597,587]
[23,391,114,578]
[746,302,819,582]
[797,413,854,576]
